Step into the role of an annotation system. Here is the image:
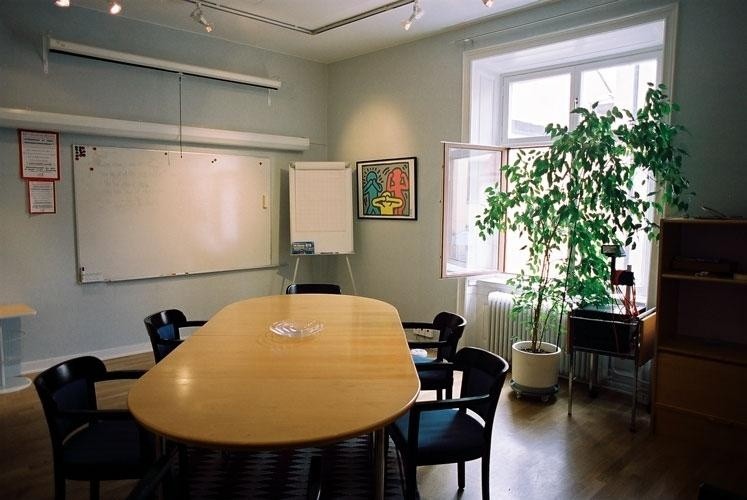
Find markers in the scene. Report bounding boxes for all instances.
[171,272,188,275]
[320,251,339,254]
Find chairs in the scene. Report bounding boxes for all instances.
[143,308,208,366]
[32,355,181,500]
[391,346,509,500]
[402,310,467,400]
[285,284,342,294]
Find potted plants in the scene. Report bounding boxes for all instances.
[475,80,696,403]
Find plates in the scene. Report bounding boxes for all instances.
[269,316,328,340]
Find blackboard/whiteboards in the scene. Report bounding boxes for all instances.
[71,143,273,284]
[288,160,356,255]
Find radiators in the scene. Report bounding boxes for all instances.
[486,291,615,390]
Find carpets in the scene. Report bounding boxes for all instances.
[126,432,406,500]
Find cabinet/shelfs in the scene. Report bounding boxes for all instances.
[650,218,747,436]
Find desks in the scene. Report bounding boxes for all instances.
[564,300,657,432]
[126,293,422,499]
[0,303,37,394]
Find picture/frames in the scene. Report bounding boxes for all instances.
[356,157,418,222]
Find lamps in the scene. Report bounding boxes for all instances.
[53,0,70,7]
[401,0,423,31]
[43,34,282,90]
[190,2,214,33]
[108,0,122,15]
[600,245,627,300]
[482,0,493,8]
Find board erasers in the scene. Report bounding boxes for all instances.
[263,195,268,209]
